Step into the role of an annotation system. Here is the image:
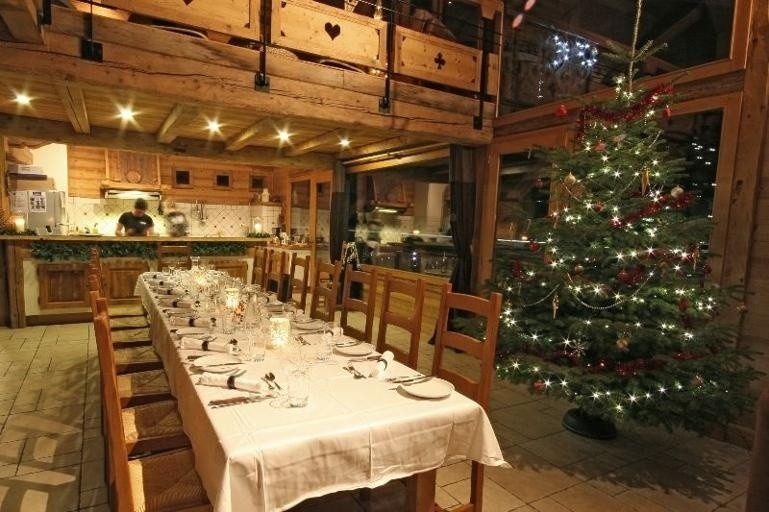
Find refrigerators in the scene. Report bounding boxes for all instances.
[8,189,65,236]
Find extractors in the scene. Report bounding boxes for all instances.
[105,190,162,201]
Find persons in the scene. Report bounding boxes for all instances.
[113,197,155,238]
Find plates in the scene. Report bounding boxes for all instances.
[144,255,456,410]
[79,233,104,237]
